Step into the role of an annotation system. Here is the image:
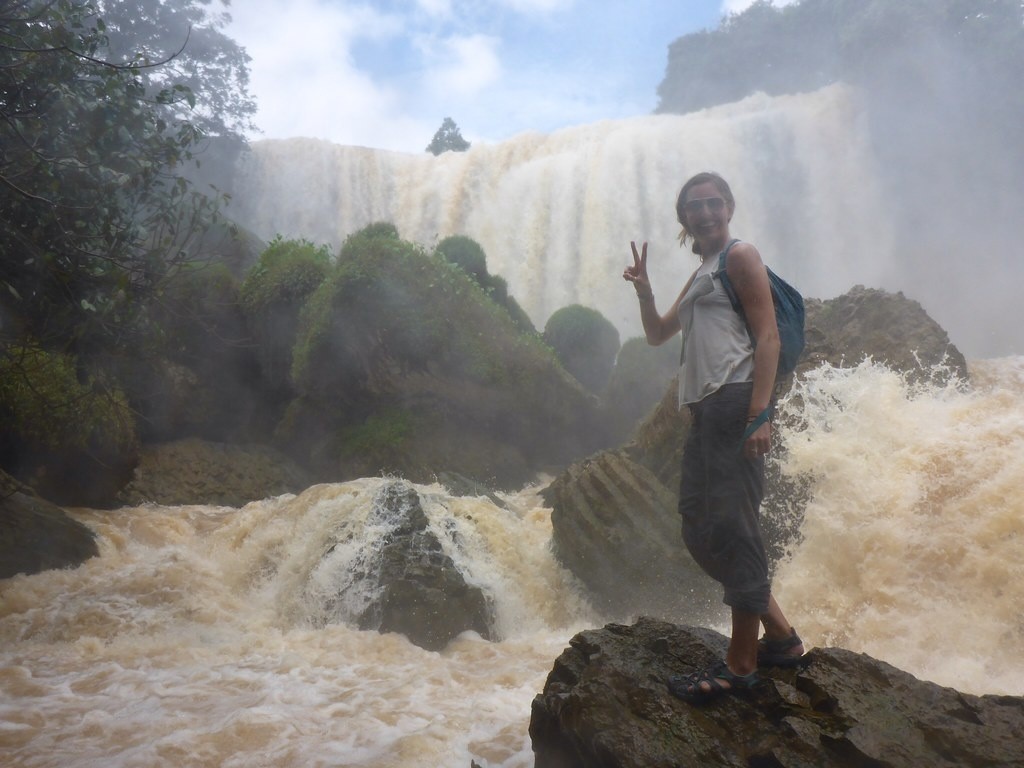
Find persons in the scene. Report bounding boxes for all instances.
[622,172,808,705]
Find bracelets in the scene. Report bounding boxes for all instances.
[744,414,769,425]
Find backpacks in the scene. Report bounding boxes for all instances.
[713,238,804,374]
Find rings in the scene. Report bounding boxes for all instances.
[633,276,637,281]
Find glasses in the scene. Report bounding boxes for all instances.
[681,197,728,212]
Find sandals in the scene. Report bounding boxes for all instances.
[755,626,803,667]
[669,660,762,702]
